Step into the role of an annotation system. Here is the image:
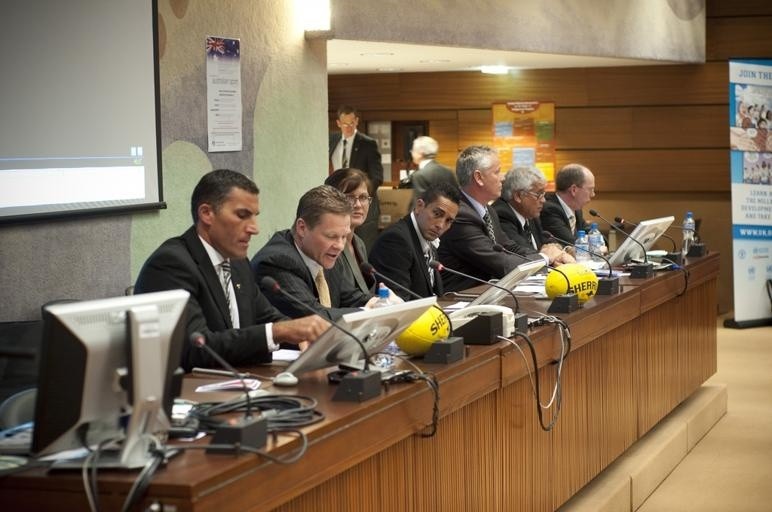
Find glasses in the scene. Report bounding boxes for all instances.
[344,194,373,206]
[518,188,546,200]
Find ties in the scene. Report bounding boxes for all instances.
[425,244,434,283]
[482,211,497,244]
[220,260,234,323]
[314,267,333,311]
[340,139,349,168]
[523,221,535,251]
[568,215,577,236]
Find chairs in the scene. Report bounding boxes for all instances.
[1,386,36,432]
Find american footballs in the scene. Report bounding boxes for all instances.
[395,302,453,355]
[546,262,598,302]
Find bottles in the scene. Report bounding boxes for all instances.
[681,212,695,257]
[575,230,589,264]
[374,288,395,368]
[588,222,604,264]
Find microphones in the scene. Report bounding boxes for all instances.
[186,331,267,448]
[428,261,529,344]
[467,202,705,312]
[260,273,381,402]
[359,261,463,364]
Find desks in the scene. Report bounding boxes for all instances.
[0,246,722,511]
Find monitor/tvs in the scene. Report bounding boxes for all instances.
[31,287,193,470]
[283,293,437,384]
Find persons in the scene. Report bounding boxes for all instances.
[407,134,459,209]
[541,162,609,249]
[368,179,460,303]
[133,169,333,372]
[328,106,383,260]
[251,182,406,326]
[439,143,563,294]
[318,169,377,296]
[494,161,576,269]
